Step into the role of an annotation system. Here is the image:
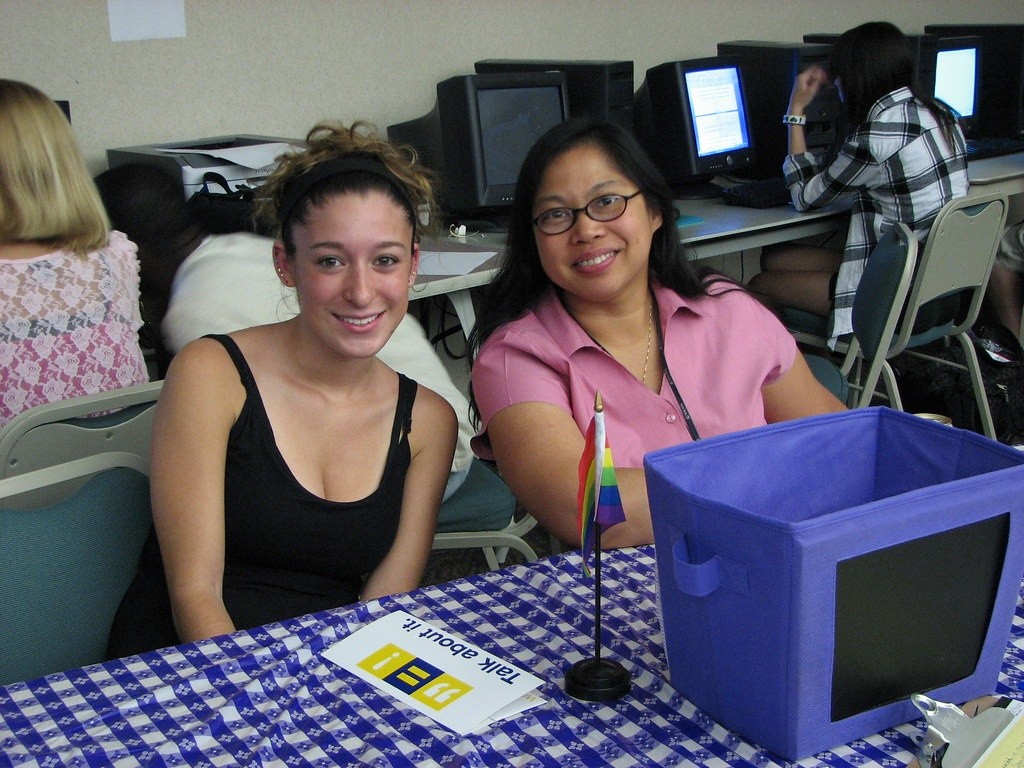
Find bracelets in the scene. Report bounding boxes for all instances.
[783,115,807,125]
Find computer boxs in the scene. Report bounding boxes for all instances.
[717,34,941,171]
[475,59,645,138]
[923,23,1024,138]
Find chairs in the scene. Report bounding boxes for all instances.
[432,455,539,571]
[0,377,163,687]
[799,193,1008,441]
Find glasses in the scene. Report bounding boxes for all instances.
[533,186,644,234]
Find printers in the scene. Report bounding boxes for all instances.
[106,133,314,205]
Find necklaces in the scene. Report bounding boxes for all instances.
[644,298,653,383]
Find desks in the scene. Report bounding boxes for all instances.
[0,444,1024,768]
[138,154,1024,373]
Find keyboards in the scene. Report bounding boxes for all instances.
[964,135,1024,162]
[719,176,792,209]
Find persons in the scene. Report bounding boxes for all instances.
[742,22,970,355]
[0,79,149,433]
[90,156,482,502]
[986,219,1024,339]
[105,119,458,663]
[465,115,850,553]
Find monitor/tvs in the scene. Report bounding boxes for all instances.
[386,70,571,228]
[935,36,986,135]
[634,56,754,198]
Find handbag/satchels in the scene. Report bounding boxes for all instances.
[894,316,1024,443]
[130,172,253,348]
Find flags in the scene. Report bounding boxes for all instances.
[578,410,625,581]
